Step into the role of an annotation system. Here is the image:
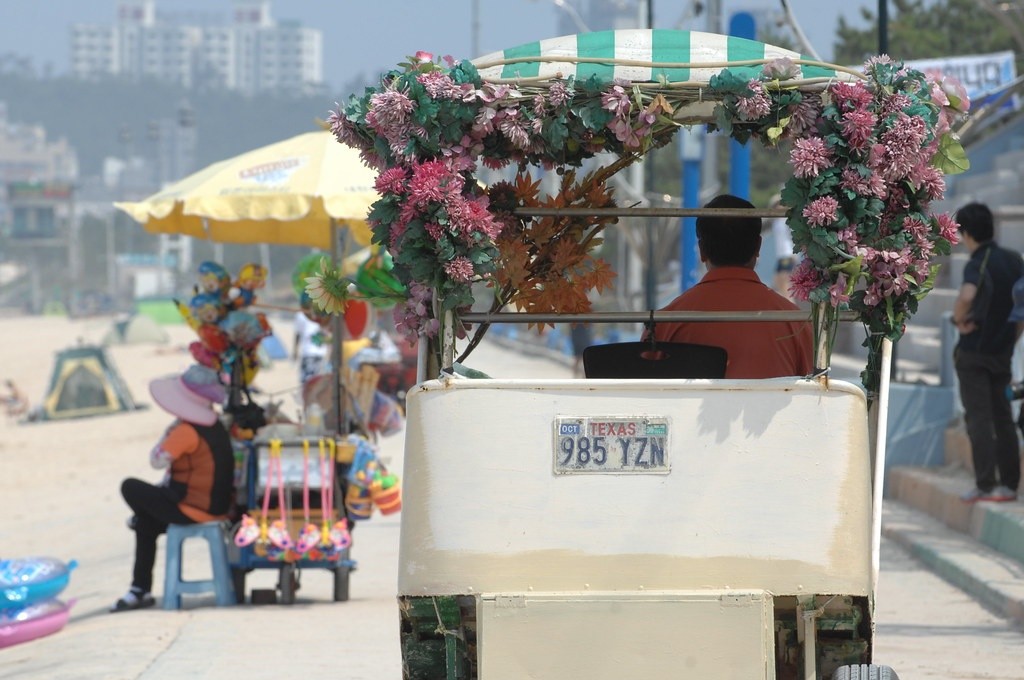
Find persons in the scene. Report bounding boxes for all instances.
[0,379,18,404]
[401,332,418,412]
[953,202,1024,502]
[109,365,235,611]
[293,311,330,417]
[775,198,796,304]
[641,195,814,379]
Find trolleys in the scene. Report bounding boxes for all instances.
[233,434,358,601]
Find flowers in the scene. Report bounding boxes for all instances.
[325,51,972,411]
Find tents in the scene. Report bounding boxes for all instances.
[104,298,184,346]
[35,342,136,422]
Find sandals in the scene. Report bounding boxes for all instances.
[110,589,155,612]
[127,515,166,533]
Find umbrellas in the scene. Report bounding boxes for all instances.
[113,128,490,442]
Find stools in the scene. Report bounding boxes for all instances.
[160,519,239,609]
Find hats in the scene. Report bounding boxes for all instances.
[148,364,225,427]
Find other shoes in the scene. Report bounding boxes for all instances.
[960,486,1017,503]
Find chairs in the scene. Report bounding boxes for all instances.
[582,340,728,380]
